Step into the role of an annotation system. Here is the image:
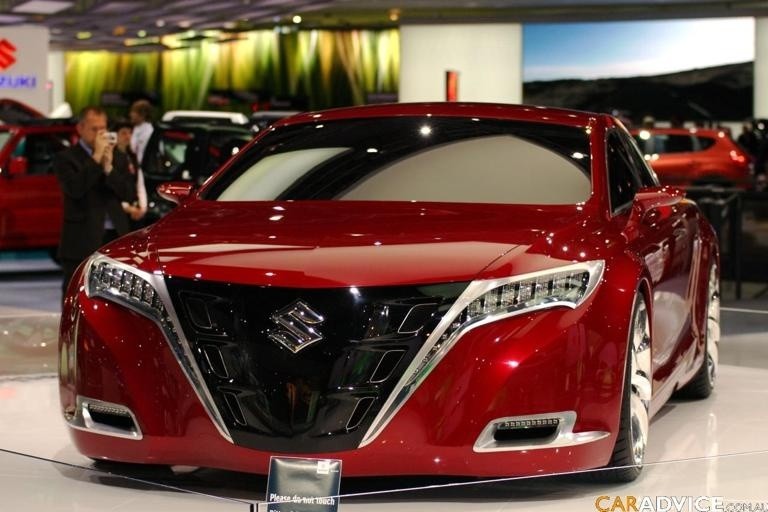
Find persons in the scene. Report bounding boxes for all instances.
[127,99,160,164]
[735,121,760,191]
[53,104,141,319]
[641,114,657,129]
[107,123,149,219]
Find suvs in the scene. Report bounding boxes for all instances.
[629,125,752,201]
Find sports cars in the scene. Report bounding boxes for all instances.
[58,104,722,484]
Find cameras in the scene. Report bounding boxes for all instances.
[105,132,118,144]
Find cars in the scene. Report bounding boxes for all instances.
[127,109,305,227]
[0,118,81,248]
